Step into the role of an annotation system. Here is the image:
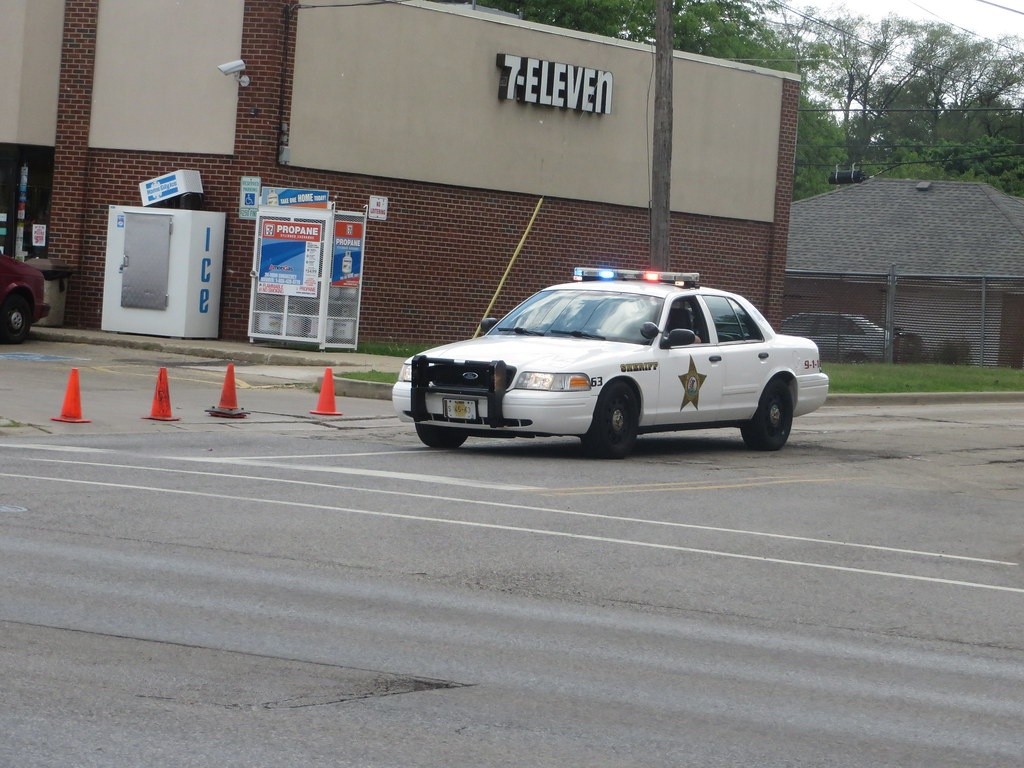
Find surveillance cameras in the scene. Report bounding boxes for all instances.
[217,59,245,80]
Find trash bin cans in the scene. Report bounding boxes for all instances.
[23,258,72,327]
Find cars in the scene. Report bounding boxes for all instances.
[778,313,897,366]
[391,267,830,456]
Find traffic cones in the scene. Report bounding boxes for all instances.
[205,363,250,419]
[51,368,91,424]
[311,367,344,416]
[140,366,181,422]
